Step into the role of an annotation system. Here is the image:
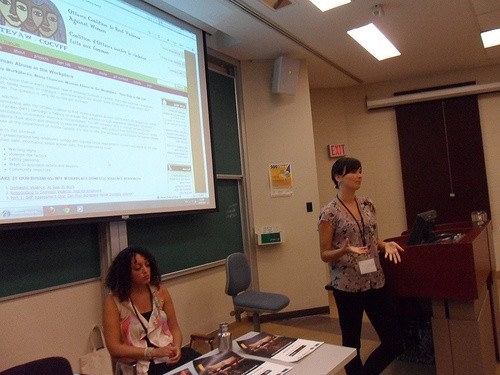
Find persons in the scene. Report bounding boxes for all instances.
[316,158,405,375]
[101,246,201,375]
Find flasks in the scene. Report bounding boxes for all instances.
[218,323,232,353]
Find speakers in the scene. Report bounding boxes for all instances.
[272,56,300,95]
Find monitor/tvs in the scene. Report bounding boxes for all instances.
[405,209,437,246]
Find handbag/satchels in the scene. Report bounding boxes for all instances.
[78,325,114,375]
[393,296,436,364]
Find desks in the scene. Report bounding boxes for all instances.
[162,330,358,375]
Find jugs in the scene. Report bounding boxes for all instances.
[471,211,486,228]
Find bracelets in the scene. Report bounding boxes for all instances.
[143,347,154,361]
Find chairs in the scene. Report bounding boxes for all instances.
[224,252,290,333]
[114,333,215,375]
[0,356,75,375]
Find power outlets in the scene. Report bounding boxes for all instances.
[306,201,314,213]
